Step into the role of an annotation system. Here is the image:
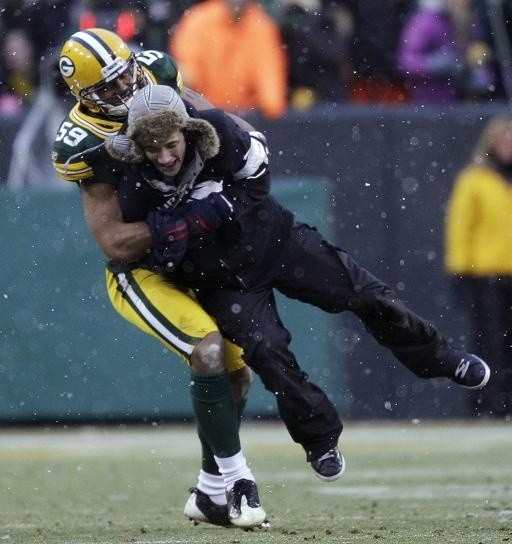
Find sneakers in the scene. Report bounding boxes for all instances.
[436,344,491,392]
[310,446,345,482]
[184,478,270,534]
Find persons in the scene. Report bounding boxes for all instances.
[0,1,287,187]
[439,115,512,418]
[49,24,274,529]
[123,82,491,481]
[277,0,504,104]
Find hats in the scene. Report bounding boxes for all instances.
[106,85,221,164]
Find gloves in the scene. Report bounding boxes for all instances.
[142,191,235,271]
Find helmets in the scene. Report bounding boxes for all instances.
[60,28,148,115]
